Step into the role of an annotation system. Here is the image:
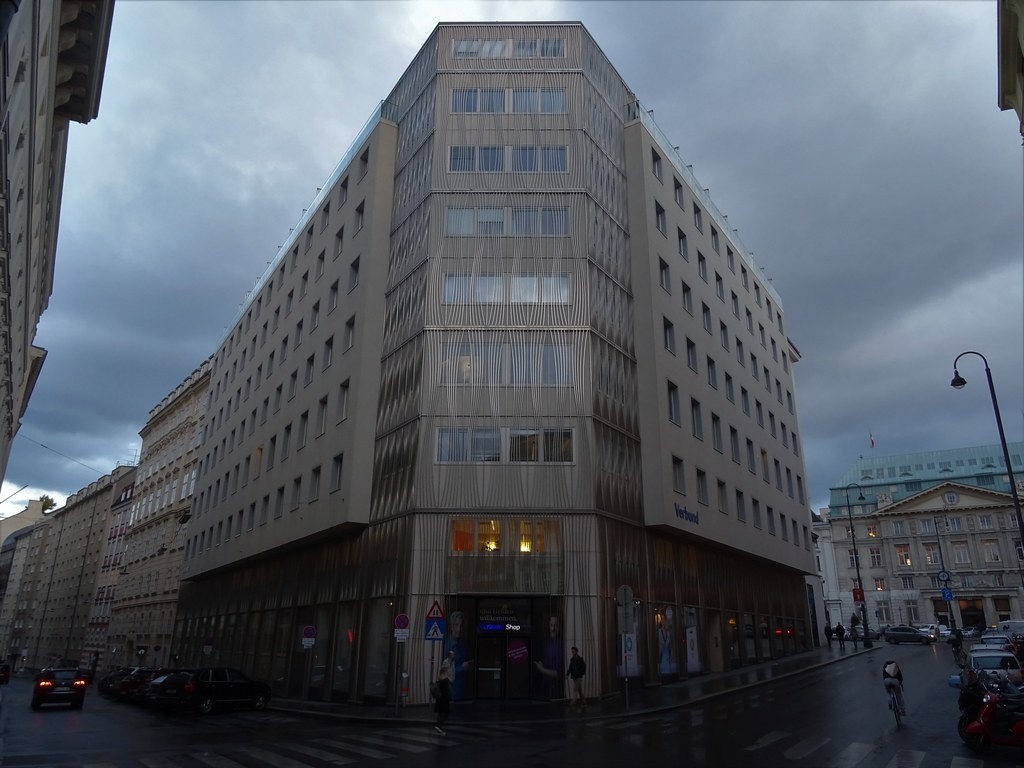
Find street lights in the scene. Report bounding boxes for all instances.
[844,482,873,644]
[933,515,958,633]
[949,351,1024,552]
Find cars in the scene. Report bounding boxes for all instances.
[30,666,88,710]
[831,620,946,641]
[151,666,273,714]
[940,628,963,637]
[957,653,1024,695]
[961,627,980,639]
[275,664,344,697]
[98,666,180,702]
[967,617,1024,659]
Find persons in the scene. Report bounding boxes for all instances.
[434,651,455,736]
[825,612,859,649]
[950,629,964,652]
[565,647,586,713]
[882,660,907,716]
[444,611,470,699]
[534,616,565,699]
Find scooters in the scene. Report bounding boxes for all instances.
[950,661,1024,749]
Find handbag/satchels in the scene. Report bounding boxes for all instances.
[885,663,899,677]
[950,635,956,639]
[432,688,441,698]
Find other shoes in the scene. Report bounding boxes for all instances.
[889,704,892,709]
[436,726,447,735]
[581,705,587,708]
[902,712,905,715]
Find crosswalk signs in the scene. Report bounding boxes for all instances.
[424,620,445,640]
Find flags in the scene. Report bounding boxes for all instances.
[869,433,875,447]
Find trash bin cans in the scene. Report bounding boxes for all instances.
[400,670,409,697]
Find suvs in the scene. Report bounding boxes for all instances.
[884,626,937,646]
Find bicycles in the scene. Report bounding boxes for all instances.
[952,644,962,661]
[888,685,905,725]
